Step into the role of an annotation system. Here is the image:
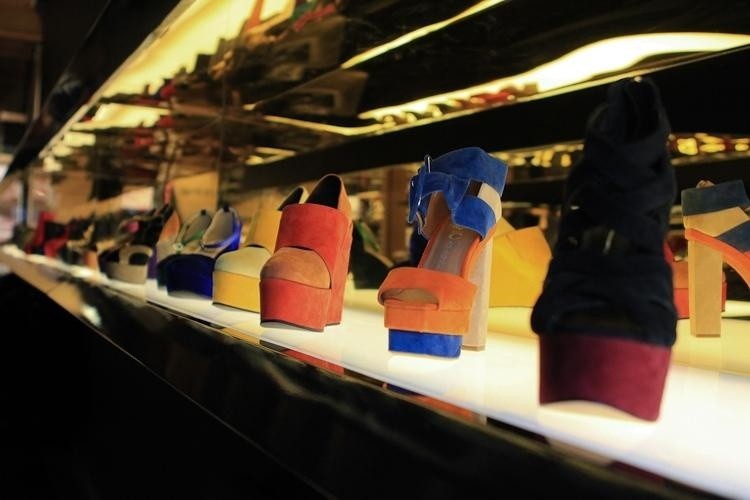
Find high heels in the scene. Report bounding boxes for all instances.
[259,173,353,331]
[23,201,241,298]
[532,80,677,420]
[211,186,313,312]
[379,147,508,359]
